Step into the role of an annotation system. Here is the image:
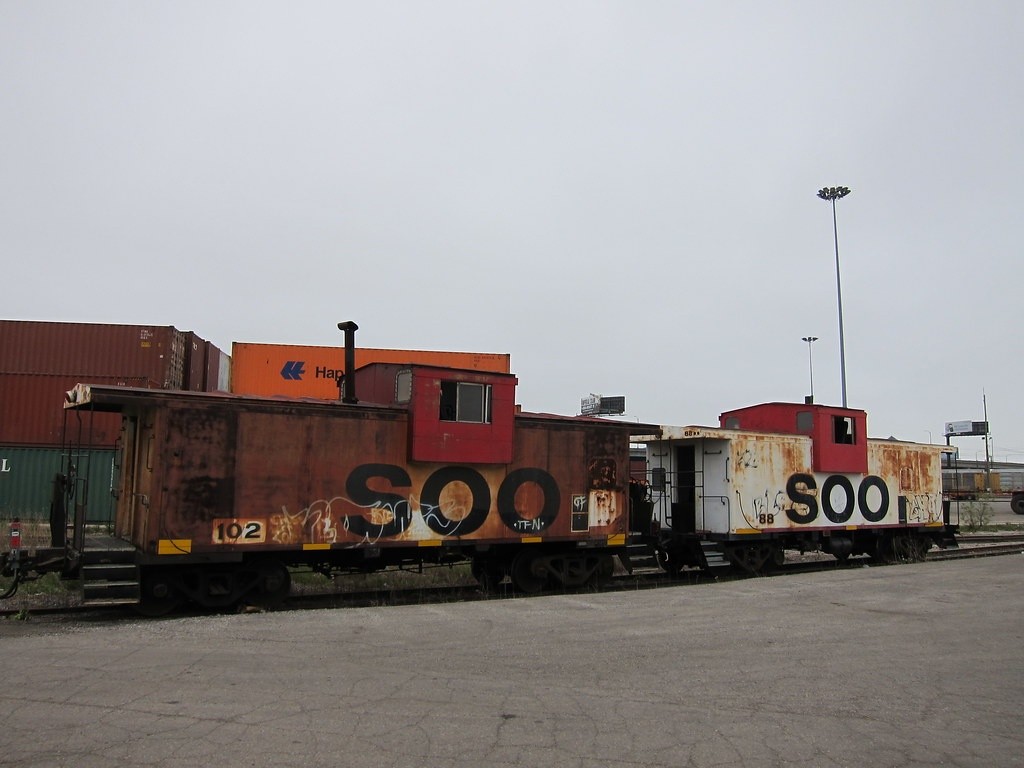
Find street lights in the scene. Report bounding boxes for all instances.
[816,186,851,408]
[801,337,819,404]
